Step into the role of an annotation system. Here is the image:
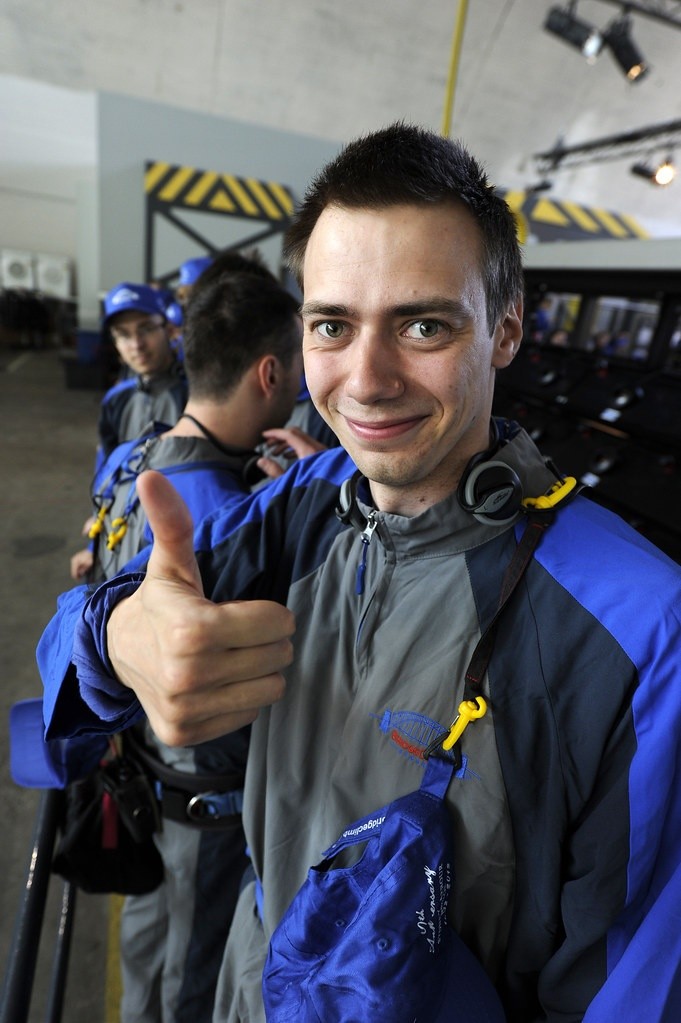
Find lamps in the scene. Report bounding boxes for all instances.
[542,0,602,58]
[633,150,676,185]
[597,2,650,82]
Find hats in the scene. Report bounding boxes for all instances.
[99,282,166,334]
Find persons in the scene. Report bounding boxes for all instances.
[70,255,341,580]
[80,277,328,1023]
[33,119,681,1023]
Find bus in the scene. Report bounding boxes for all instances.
[487,258,681,569]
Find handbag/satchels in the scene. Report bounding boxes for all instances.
[262,792,505,1022]
